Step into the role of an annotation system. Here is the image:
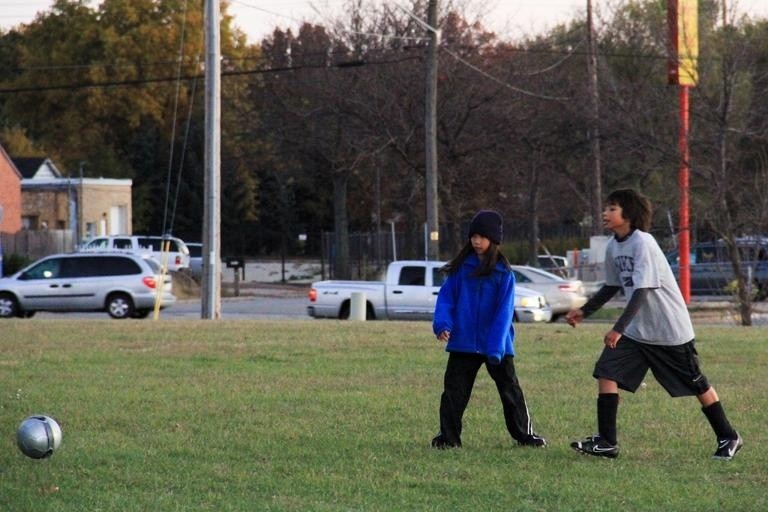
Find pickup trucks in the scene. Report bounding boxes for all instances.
[307,260,552,321]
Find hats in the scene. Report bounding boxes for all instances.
[468,210,503,245]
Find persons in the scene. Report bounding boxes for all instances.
[564,188,745,461]
[431,209,547,448]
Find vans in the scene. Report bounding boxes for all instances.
[83,236,203,272]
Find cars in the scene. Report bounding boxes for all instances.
[0,253,176,318]
[510,265,586,322]
[666,240,768,301]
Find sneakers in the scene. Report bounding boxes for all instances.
[711,431,743,460]
[570,435,619,460]
[432,436,462,449]
[518,436,546,446]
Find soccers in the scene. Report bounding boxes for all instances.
[16,415,62,459]
